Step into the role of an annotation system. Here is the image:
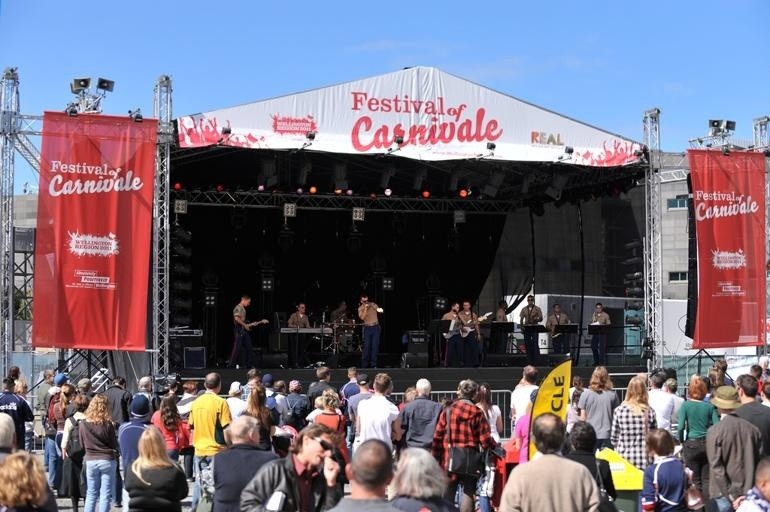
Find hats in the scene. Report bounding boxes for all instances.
[166,372,181,386]
[665,378,678,389]
[48,373,92,394]
[262,373,303,387]
[709,384,743,409]
[129,394,150,417]
[357,373,369,385]
[230,381,243,395]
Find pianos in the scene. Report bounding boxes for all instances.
[279,328,333,334]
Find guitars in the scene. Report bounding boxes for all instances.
[238,319,269,335]
[460,312,493,338]
[442,322,472,339]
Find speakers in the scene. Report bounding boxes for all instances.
[255,351,288,369]
[486,354,510,366]
[683,171,698,339]
[605,307,624,353]
[401,353,428,368]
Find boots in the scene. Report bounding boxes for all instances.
[71,494,79,512]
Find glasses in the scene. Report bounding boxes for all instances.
[312,434,331,453]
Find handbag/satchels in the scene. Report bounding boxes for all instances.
[448,446,484,477]
[683,482,706,510]
[42,410,57,438]
[198,461,216,502]
[596,485,618,512]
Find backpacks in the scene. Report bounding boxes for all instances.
[66,416,84,461]
[284,397,301,422]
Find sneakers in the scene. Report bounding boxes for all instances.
[115,501,124,509]
[185,475,195,482]
[226,362,240,369]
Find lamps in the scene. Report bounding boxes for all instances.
[633,150,645,164]
[481,142,496,159]
[301,131,315,149]
[560,146,573,161]
[216,127,231,146]
[386,137,403,154]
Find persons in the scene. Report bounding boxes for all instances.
[38,367,600,512]
[287,302,313,352]
[228,295,257,368]
[357,293,385,368]
[591,303,611,366]
[440,302,464,368]
[565,360,769,512]
[546,304,570,356]
[520,296,542,366]
[495,301,507,354]
[330,300,353,323]
[459,301,481,368]
[1,366,58,511]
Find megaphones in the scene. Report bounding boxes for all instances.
[74,78,91,89]
[70,82,82,94]
[97,77,114,92]
[724,120,736,131]
[709,119,724,129]
